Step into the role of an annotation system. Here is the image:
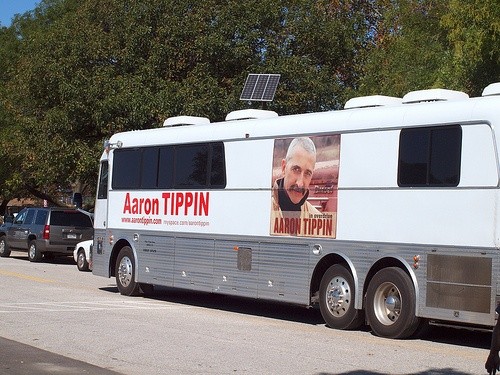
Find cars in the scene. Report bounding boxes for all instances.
[73,239,94,271]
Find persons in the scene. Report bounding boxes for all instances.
[485,304,500,375]
[272,137,326,236]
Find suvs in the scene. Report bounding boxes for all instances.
[0,207,94,263]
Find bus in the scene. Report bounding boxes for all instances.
[90,81,500,341]
